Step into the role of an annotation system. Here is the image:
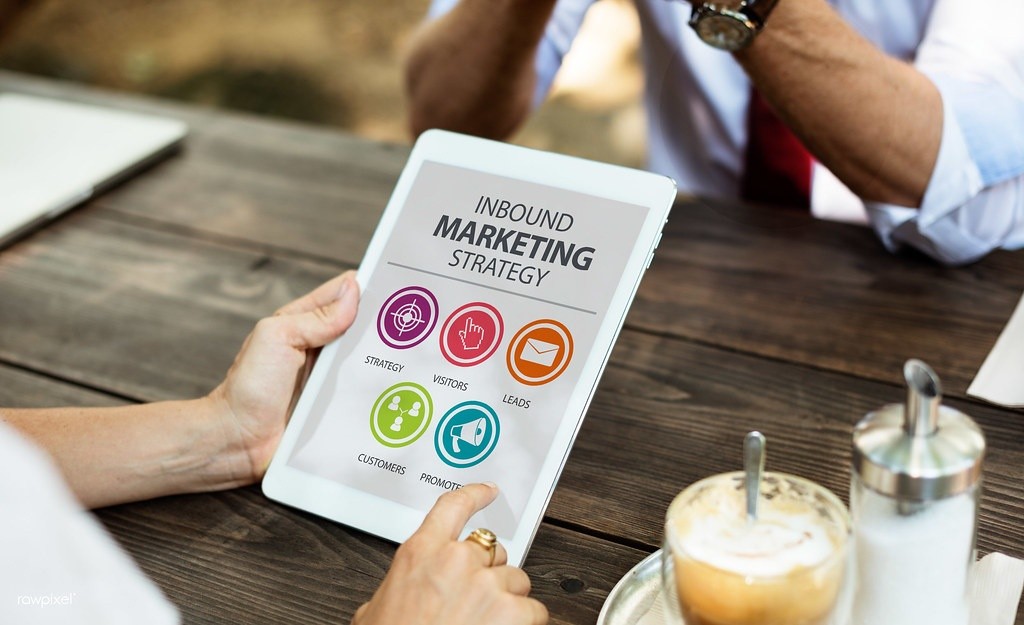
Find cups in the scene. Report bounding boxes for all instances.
[660,470,857,625]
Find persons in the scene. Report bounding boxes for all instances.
[1,265,554,625]
[399,0,1024,267]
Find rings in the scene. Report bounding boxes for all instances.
[465,527,498,568]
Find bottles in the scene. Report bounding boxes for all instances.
[849,359,986,625]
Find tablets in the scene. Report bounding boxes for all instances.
[266,129,677,567]
[0,92,189,250]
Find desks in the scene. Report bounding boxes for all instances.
[0,71,1024,625]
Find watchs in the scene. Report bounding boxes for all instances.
[687,0,783,52]
